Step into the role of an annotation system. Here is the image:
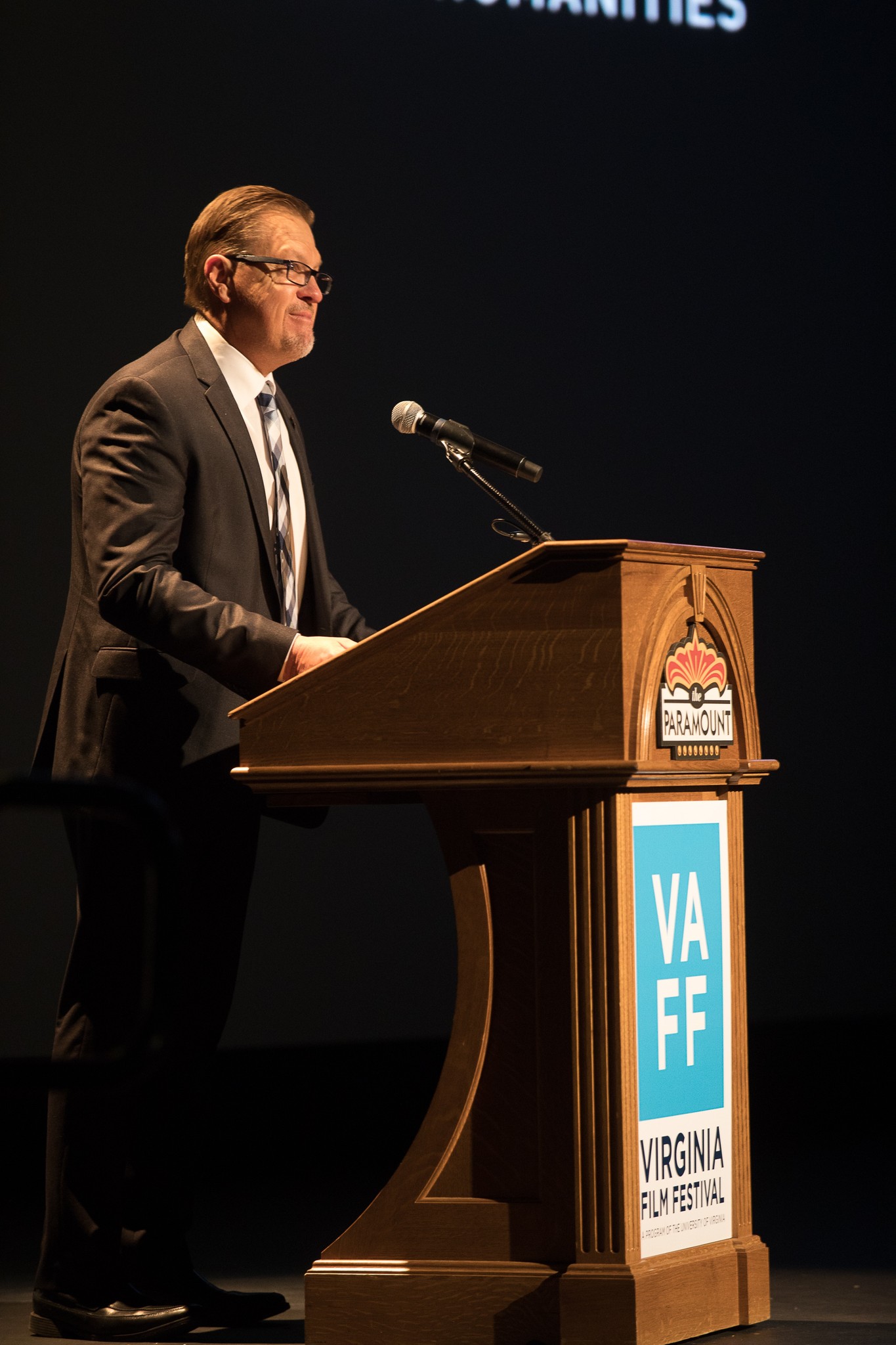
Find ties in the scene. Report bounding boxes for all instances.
[255,383,298,630]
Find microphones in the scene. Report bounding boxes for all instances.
[391,400,547,483]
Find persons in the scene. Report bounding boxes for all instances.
[19,183,383,1344]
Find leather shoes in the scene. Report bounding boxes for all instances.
[141,1267,292,1332]
[31,1269,189,1339]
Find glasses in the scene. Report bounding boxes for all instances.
[231,254,332,295]
